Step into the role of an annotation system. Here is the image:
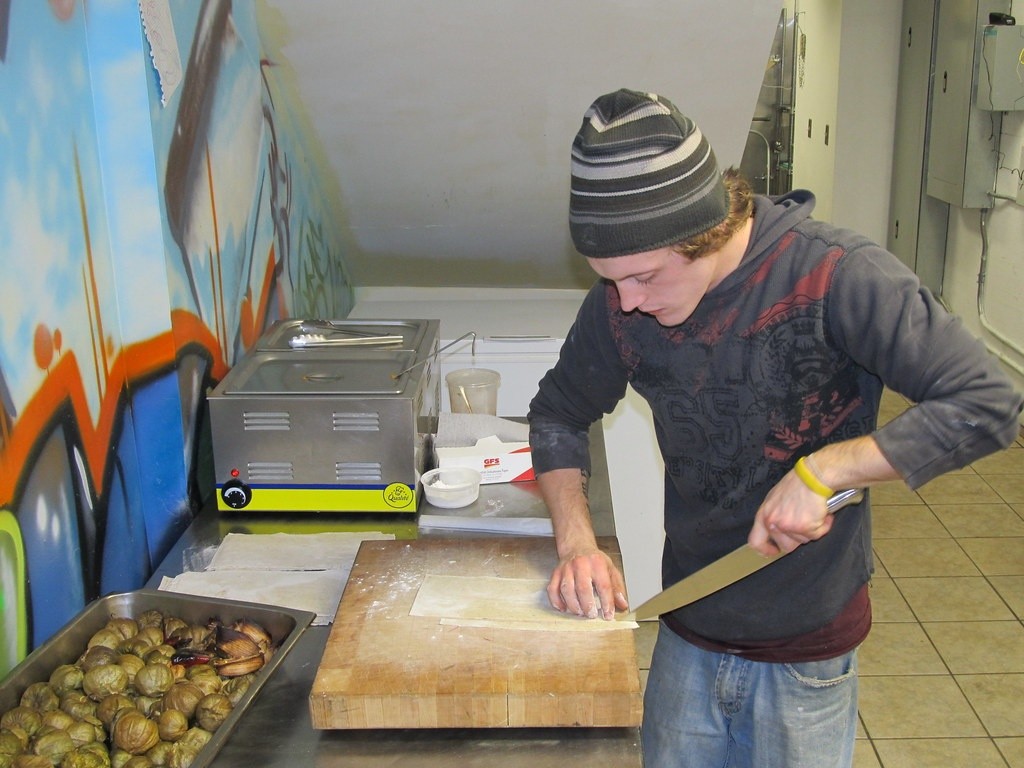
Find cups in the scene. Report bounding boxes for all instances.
[445,368,501,417]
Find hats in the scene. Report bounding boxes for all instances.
[568,87,730,259]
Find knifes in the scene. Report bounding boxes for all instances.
[618,489,865,622]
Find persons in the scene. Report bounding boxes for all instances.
[526,87,1023,768]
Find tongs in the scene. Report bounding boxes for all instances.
[288,318,404,348]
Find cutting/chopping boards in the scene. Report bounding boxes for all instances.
[308,536,644,731]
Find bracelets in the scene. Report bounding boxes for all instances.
[794,456,836,499]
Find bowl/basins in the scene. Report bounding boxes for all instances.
[420,466,483,509]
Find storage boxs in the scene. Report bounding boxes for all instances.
[435,410,535,485]
